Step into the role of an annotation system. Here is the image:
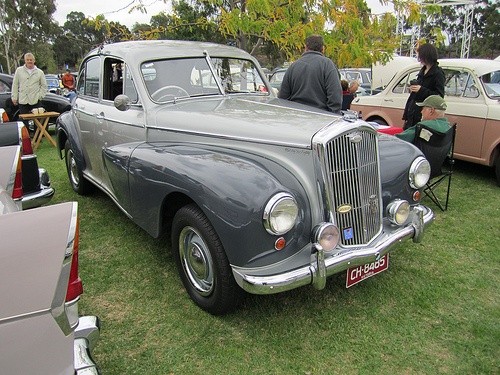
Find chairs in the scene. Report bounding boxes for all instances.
[412,123,457,212]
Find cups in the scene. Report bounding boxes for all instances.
[31,108,38,114]
[39,108,45,113]
[410,80,418,92]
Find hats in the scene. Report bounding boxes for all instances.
[415,94,447,111]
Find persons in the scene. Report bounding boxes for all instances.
[402,43,446,131]
[394,95,452,144]
[10,53,48,144]
[341,80,360,112]
[62,70,77,94]
[278,36,343,115]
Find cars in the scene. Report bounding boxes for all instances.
[45,74,65,95]
[0,73,72,130]
[196,66,381,97]
[349,58,500,188]
[55,39,437,313]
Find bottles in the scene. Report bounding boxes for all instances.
[359,111,362,119]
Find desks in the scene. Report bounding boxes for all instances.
[19,112,60,154]
